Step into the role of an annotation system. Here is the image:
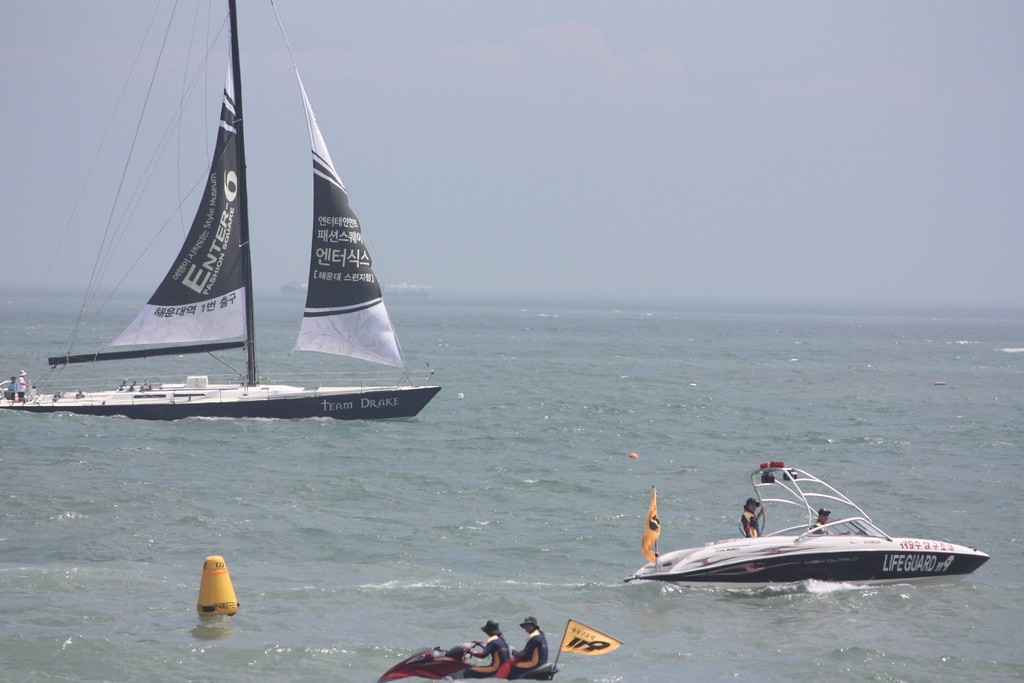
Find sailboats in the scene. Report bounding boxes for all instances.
[1,1,444,418]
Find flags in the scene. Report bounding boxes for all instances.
[560,619,624,655]
[641,487,660,563]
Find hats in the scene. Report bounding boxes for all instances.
[819,508,831,514]
[520,616,539,629]
[18,370,27,377]
[744,498,761,509]
[480,620,502,635]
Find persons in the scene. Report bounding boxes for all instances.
[17,370,28,402]
[507,617,547,679]
[464,620,510,678]
[8,376,18,400]
[741,498,765,538]
[812,508,831,536]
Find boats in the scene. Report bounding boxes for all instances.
[618,459,989,584]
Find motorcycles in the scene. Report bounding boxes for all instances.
[374,640,559,682]
